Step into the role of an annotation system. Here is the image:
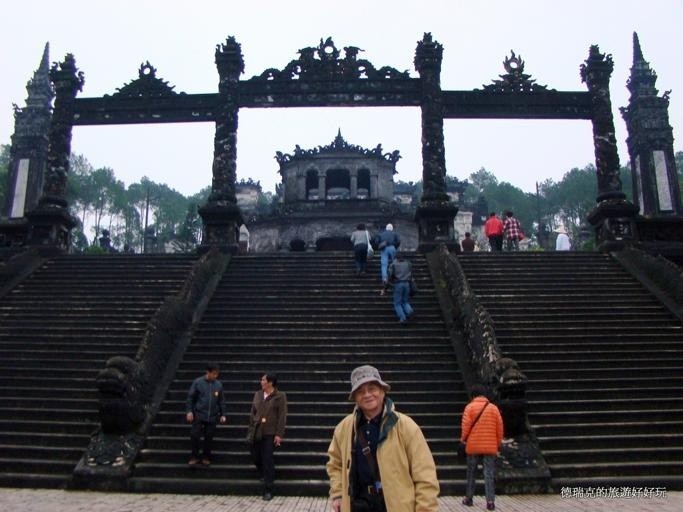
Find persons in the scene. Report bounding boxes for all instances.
[99,229,137,254]
[350,221,415,323]
[184,362,228,467]
[244,374,289,501]
[459,384,505,510]
[485,211,521,252]
[325,363,441,512]
[461,231,476,254]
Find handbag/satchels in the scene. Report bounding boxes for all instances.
[409,277,418,290]
[367,241,374,260]
[457,442,466,461]
[246,415,262,445]
[378,240,388,250]
[518,231,525,240]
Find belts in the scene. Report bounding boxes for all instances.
[356,485,384,497]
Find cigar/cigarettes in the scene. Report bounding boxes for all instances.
[278,443,281,446]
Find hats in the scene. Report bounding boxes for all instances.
[553,226,566,233]
[348,365,391,402]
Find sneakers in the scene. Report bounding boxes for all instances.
[462,497,473,506]
[263,491,274,501]
[486,501,496,511]
[202,460,210,465]
[188,457,200,464]
